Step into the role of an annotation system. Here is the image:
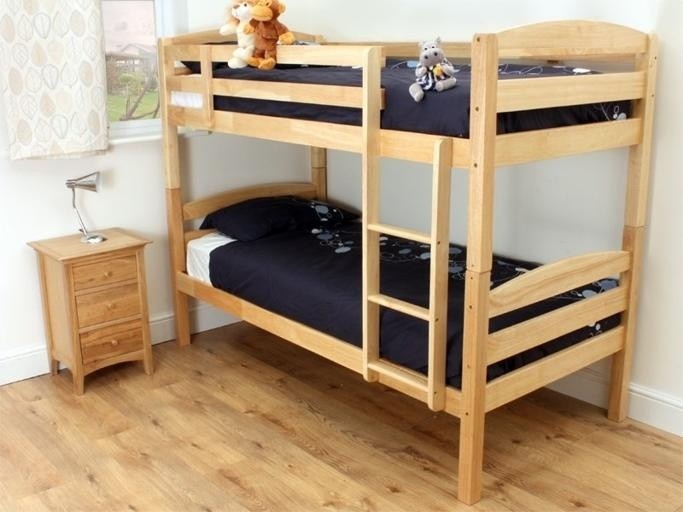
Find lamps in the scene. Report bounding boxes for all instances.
[66,171,108,244]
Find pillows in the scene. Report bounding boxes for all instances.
[180,41,328,74]
[199,196,360,243]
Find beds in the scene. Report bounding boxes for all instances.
[158,19,660,504]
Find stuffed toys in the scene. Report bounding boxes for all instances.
[217,0,260,71]
[241,1,294,72]
[408,38,458,103]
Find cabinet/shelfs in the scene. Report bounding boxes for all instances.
[27,228,155,395]
